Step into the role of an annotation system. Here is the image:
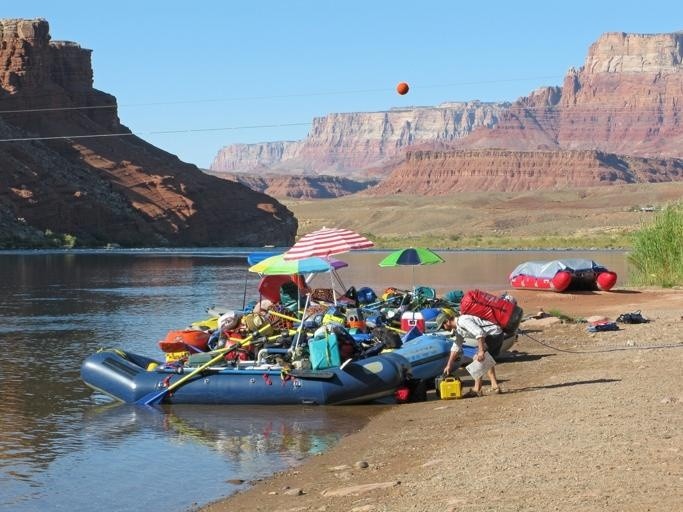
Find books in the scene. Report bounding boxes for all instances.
[465,350,496,381]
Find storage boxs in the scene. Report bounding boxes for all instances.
[401,311,425,333]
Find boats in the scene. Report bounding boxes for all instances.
[508,259,618,293]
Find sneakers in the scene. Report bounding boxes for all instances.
[484,387,501,395]
[462,388,483,398]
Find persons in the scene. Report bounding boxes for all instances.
[435,313,503,398]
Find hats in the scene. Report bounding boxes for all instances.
[436,313,450,331]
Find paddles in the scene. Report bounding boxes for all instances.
[154,366,334,378]
[385,325,478,358]
[340,359,353,370]
[134,318,282,404]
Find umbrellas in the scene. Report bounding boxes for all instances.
[377,246,445,288]
[245,252,331,318]
[282,226,376,305]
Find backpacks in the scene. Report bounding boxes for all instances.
[457,287,524,334]
